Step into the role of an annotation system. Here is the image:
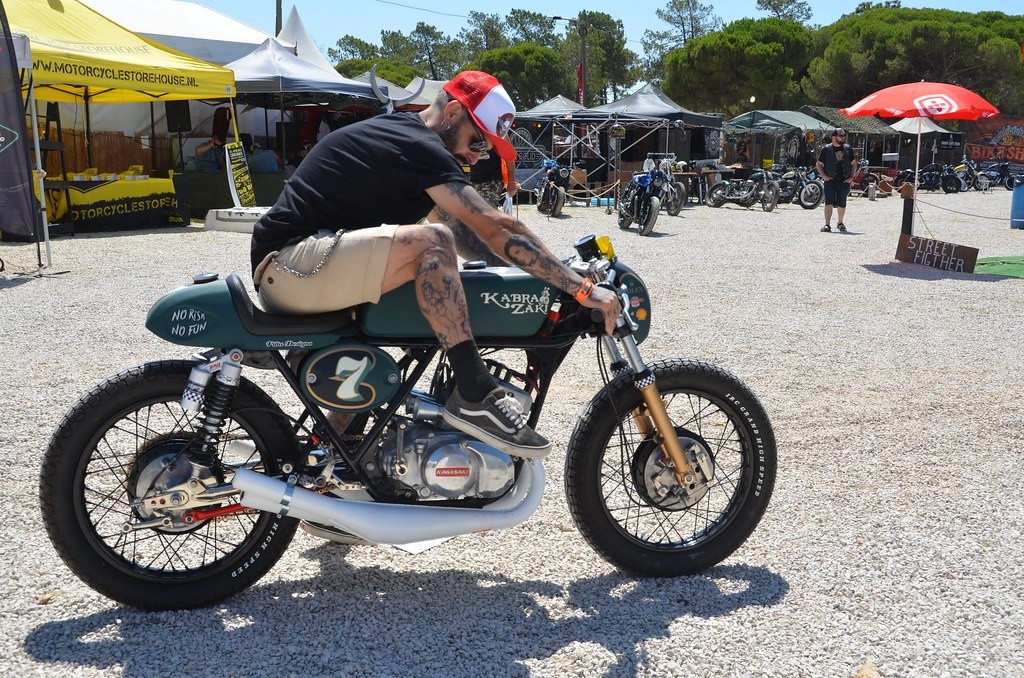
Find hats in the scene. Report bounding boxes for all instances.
[832,128,846,136]
[250,143,261,152]
[443,70,518,162]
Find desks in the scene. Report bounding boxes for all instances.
[673,172,700,206]
[44,178,183,232]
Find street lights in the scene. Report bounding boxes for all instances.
[552,16,585,159]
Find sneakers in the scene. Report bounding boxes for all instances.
[443,384,552,458]
[300,518,377,545]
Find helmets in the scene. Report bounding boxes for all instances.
[675,161,689,173]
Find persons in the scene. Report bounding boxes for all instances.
[194,135,227,174]
[247,143,285,172]
[292,139,314,169]
[816,128,857,232]
[251,70,622,546]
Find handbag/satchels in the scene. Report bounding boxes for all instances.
[502,194,513,217]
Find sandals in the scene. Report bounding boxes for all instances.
[837,222,846,232]
[820,225,831,232]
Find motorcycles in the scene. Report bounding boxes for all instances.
[649,165,826,217]
[894,153,1017,192]
[848,157,880,194]
[37,235,778,615]
[615,170,669,235]
[536,160,574,218]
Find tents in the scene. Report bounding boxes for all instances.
[517,82,723,160]
[888,115,966,139]
[0,0,463,113]
[790,104,900,170]
[722,111,835,163]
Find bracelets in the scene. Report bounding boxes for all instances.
[573,276,593,302]
[850,176,854,179]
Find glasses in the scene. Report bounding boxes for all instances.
[214,143,222,149]
[448,98,490,161]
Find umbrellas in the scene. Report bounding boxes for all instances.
[843,80,1000,236]
